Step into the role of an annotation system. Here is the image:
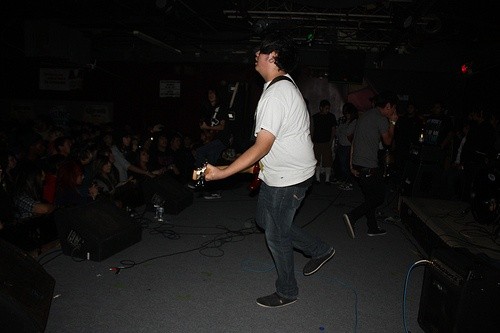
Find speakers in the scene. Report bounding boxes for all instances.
[53,200,141,263]
[147,173,193,215]
[415,245,500,333]
[205,159,247,191]
[0,240,55,333]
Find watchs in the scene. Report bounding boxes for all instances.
[388,120,397,126]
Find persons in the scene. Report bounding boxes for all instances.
[186,87,232,199]
[310,99,336,185]
[205,35,336,307]
[343,91,400,241]
[0,116,212,257]
[390,99,500,210]
[331,104,358,191]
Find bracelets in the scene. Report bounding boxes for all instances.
[146,170,148,177]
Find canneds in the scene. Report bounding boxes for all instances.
[153,205,164,221]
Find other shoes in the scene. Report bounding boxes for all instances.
[325,182,331,186]
[343,213,355,238]
[315,180,321,184]
[256,294,299,308]
[303,247,336,276]
[367,226,388,236]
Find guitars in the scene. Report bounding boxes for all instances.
[193,161,262,190]
